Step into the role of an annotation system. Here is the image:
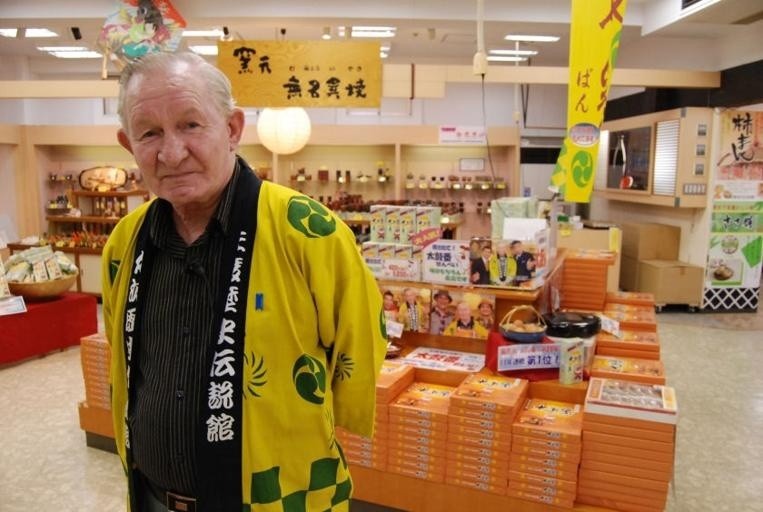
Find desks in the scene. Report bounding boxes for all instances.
[1,290,99,368]
[377,248,572,352]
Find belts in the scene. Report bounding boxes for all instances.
[144,481,197,512]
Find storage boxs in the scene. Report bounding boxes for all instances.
[491,197,559,283]
[620,220,704,310]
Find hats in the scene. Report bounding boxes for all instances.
[434,290,452,303]
[478,299,493,310]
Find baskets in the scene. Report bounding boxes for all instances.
[8,271,79,299]
[499,305,548,342]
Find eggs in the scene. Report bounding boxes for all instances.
[508,320,536,332]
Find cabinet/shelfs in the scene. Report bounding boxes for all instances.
[235,124,522,250]
[594,106,715,211]
[23,123,153,257]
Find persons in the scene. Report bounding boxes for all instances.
[487,242,517,286]
[505,241,536,284]
[472,299,494,331]
[470,240,495,284]
[481,244,493,269]
[97,49,391,511]
[377,290,400,335]
[441,300,489,341]
[398,287,427,334]
[428,289,455,337]
